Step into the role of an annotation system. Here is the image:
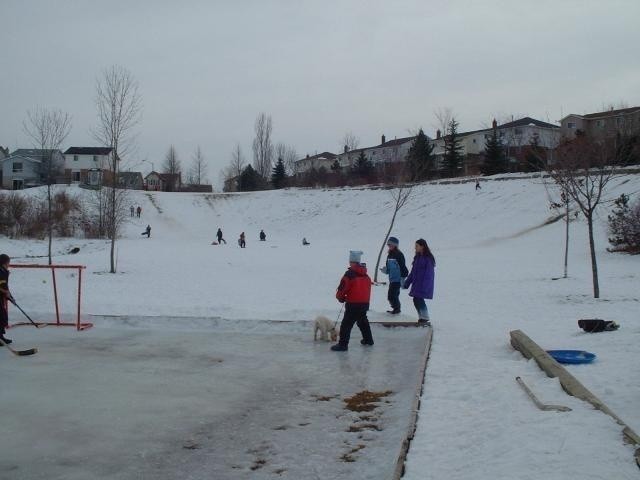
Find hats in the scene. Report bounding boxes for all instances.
[0,254,10,265]
[349,250,363,262]
[387,237,399,248]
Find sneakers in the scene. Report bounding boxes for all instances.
[418,319,429,322]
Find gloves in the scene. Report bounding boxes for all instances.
[379,267,387,273]
[401,277,406,288]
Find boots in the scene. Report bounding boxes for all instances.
[331,343,348,351]
[387,306,401,314]
[361,339,374,345]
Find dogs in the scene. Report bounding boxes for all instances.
[314,317,337,341]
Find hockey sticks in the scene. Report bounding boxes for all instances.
[0,338,39,355]
[6,298,48,328]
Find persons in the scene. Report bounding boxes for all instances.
[380,237,436,324]
[217,228,222,244]
[260,230,266,241]
[303,237,310,245]
[240,232,246,248]
[330,251,374,351]
[130,205,141,218]
[146,225,151,237]
[476,178,481,190]
[0,254,15,346]
